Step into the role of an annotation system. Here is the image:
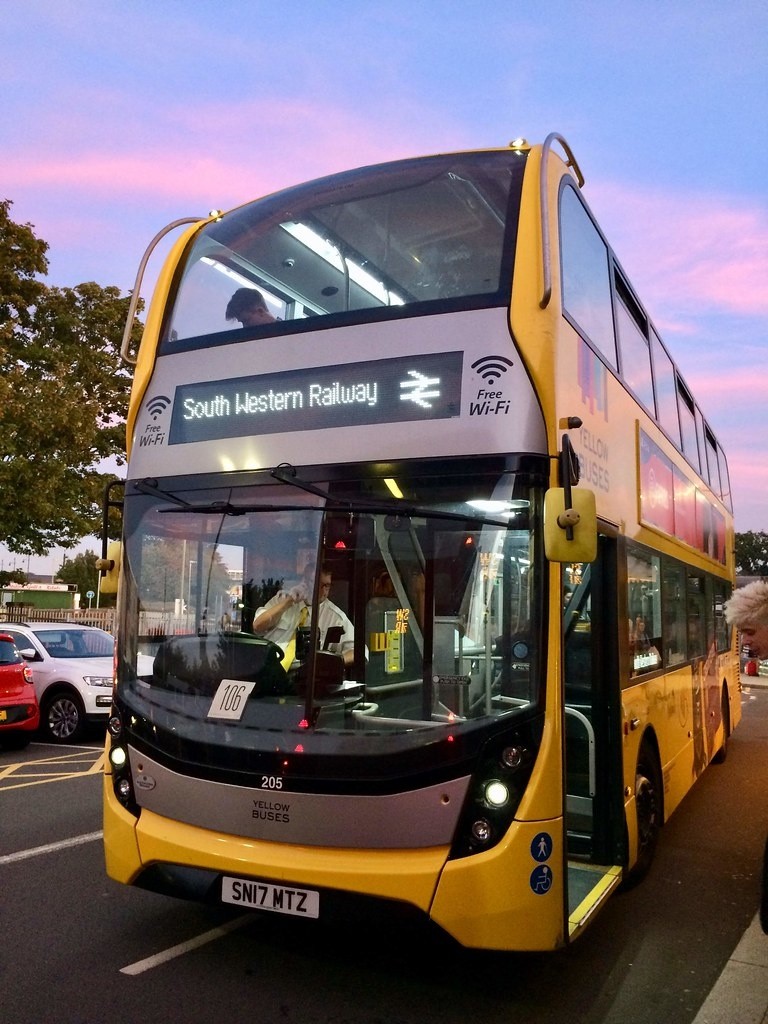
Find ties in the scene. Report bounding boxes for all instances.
[279,607,308,674]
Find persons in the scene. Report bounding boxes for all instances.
[373,567,436,632]
[225,286,284,328]
[722,579,768,661]
[620,609,732,673]
[561,584,572,612]
[251,564,371,682]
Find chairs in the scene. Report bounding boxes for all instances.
[367,594,420,656]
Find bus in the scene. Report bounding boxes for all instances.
[94,130,743,947]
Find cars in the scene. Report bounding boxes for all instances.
[0,632,40,752]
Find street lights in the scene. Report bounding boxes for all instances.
[86,590,95,610]
[187,561,197,617]
[8,558,16,572]
[22,555,30,580]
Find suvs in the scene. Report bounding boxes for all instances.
[0,622,156,746]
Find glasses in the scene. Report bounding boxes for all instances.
[308,578,332,590]
